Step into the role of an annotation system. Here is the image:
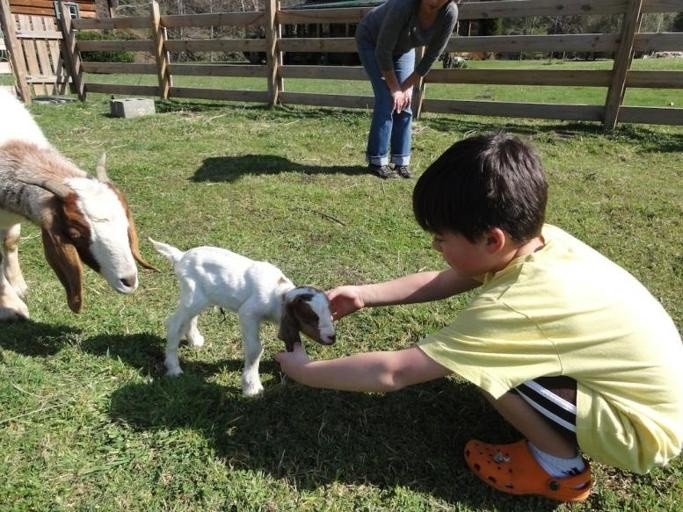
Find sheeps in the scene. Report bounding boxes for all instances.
[147,235,337,399]
[0,85,161,322]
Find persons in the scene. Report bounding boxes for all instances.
[277,128,683,503]
[355,0,459,179]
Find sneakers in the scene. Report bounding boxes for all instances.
[394,165,413,178]
[368,163,395,178]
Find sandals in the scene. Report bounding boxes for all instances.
[465,439,591,502]
